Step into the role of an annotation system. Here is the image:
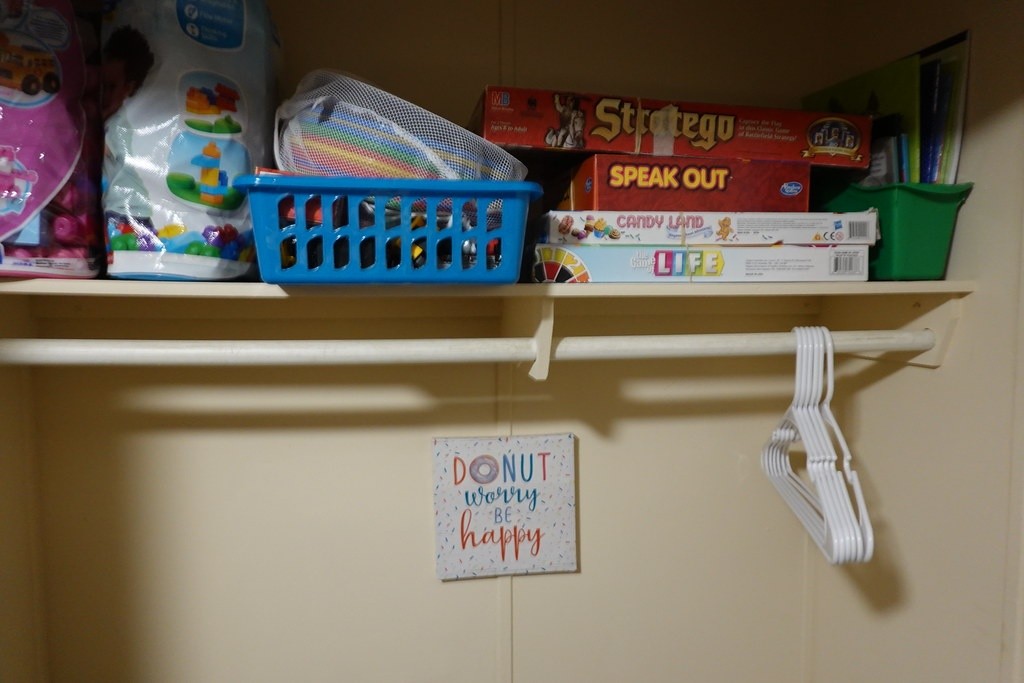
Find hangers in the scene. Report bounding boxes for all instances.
[759,325,875,566]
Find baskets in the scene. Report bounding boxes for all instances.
[231,172,545,286]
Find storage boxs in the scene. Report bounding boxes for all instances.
[465,84,880,286]
